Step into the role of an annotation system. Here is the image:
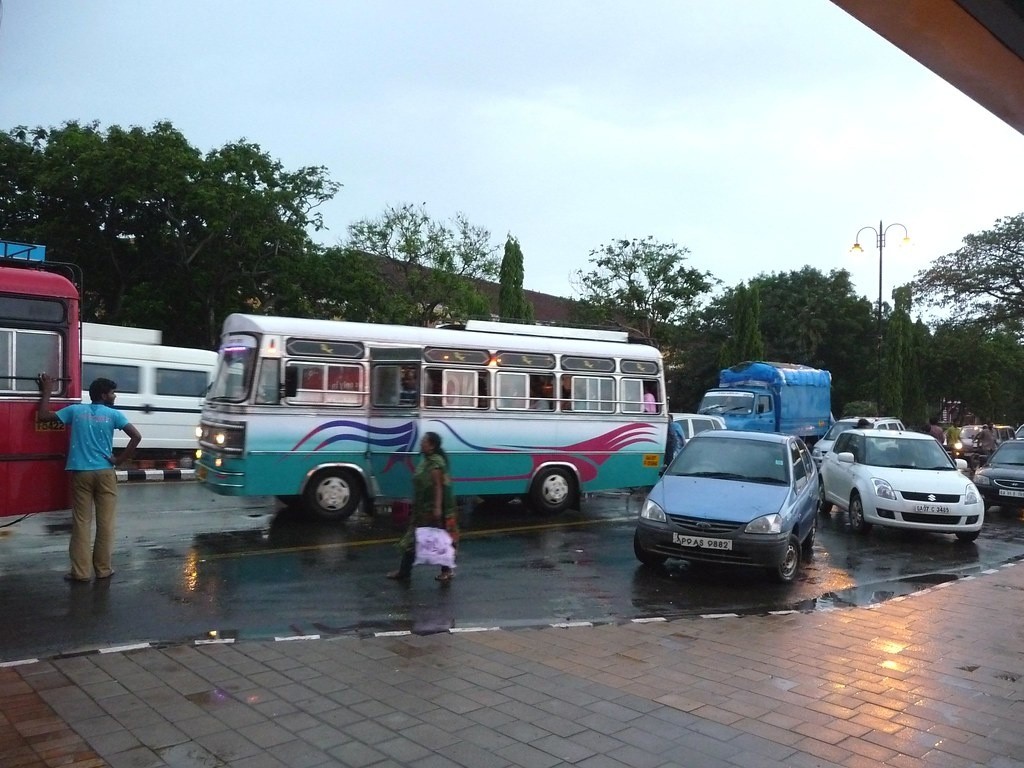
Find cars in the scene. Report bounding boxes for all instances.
[817,428,985,544]
[634,427,822,587]
[666,408,1023,518]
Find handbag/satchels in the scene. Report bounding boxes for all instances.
[411,526,457,568]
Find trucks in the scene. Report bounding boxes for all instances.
[695,360,831,449]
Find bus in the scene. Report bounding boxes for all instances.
[79,319,248,473]
[0,236,84,527]
[190,310,677,524]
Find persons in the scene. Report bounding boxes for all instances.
[920,417,945,444]
[973,421,1000,459]
[34,372,142,582]
[668,413,686,474]
[643,383,658,413]
[946,418,965,459]
[852,419,870,429]
[385,431,459,580]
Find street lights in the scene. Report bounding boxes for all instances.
[850,218,911,417]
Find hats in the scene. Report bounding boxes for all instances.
[983,424,988,426]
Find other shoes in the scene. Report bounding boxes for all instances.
[64,573,90,581]
[435,568,456,580]
[96,569,115,578]
[386,569,411,579]
